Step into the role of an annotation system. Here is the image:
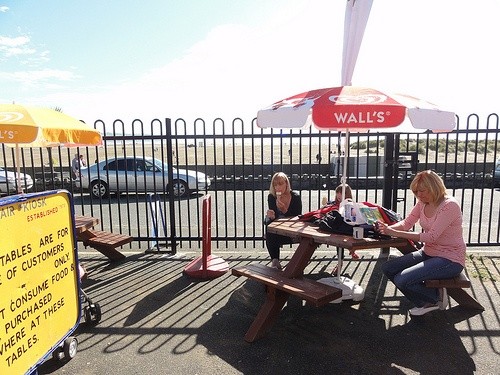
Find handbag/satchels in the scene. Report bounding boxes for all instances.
[317,211,368,238]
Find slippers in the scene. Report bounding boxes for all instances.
[409,305,440,315]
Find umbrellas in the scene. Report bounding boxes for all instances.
[257,85,456,303]
[0,102,103,194]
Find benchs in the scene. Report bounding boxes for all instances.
[425,269,471,289]
[86,229,134,250]
[231,264,343,308]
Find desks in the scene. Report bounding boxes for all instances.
[243,216,485,342]
[74,214,127,261]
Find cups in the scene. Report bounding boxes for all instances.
[353,227,364,239]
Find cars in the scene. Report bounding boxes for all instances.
[0,167,34,196]
[72,155,211,199]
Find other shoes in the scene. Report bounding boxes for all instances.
[352,253,359,259]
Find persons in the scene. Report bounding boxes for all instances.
[79,155,87,168]
[71,153,83,183]
[264,172,303,269]
[323,184,360,261]
[372,169,466,315]
